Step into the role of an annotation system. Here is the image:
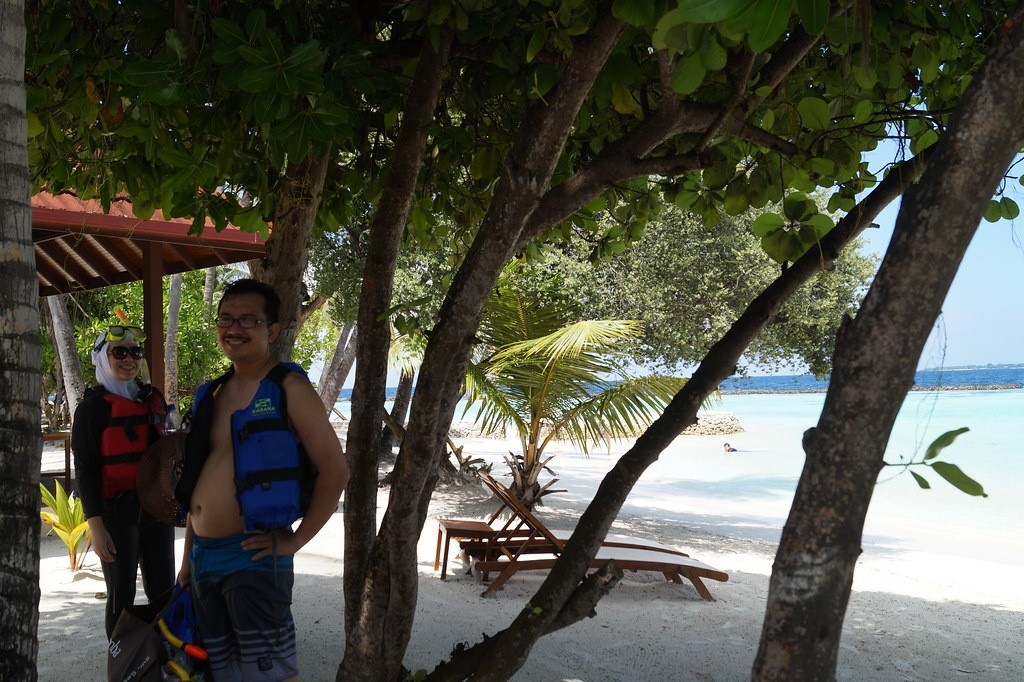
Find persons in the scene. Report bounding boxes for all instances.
[177,279,348,682]
[72,326,176,640]
[724,443,737,452]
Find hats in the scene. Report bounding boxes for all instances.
[136,431,189,528]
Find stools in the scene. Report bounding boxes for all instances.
[434,520,493,583]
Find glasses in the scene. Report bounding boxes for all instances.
[107,346,144,360]
[215,316,273,329]
[93,325,144,351]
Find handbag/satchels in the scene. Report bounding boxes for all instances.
[108,583,192,682]
[158,584,208,682]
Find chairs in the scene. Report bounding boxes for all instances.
[461,481,729,600]
[454,470,690,585]
